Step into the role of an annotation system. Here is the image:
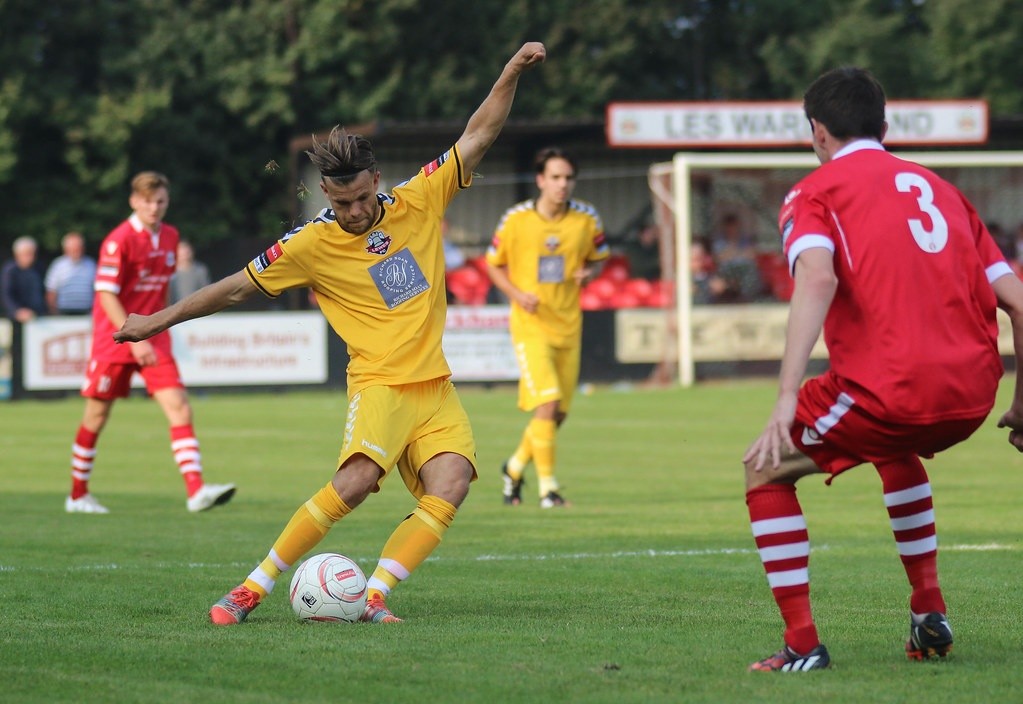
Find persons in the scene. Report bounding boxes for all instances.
[741,68,1023,671]
[114,39,547,627]
[166,240,210,306]
[0,236,45,321]
[44,232,96,315]
[64,172,237,512]
[487,148,608,504]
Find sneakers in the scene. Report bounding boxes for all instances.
[539,491,572,507]
[750,642,830,674]
[208,583,261,625]
[501,462,524,506]
[65,494,108,514]
[905,609,954,662]
[186,484,235,512]
[358,593,404,623]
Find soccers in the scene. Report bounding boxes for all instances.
[288,552,369,626]
